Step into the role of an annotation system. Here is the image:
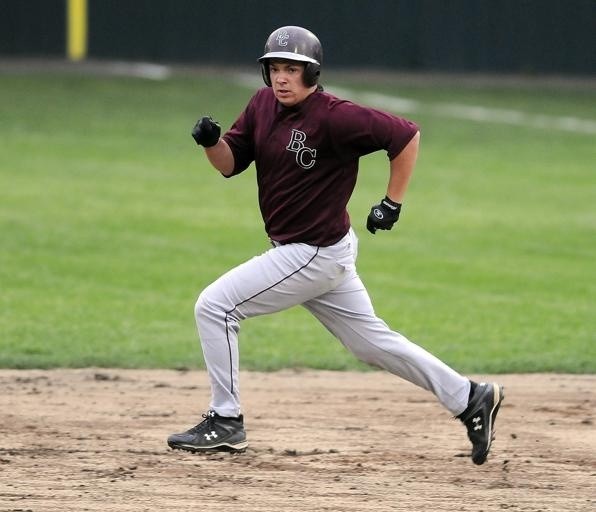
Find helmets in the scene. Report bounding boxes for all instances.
[256,23,324,70]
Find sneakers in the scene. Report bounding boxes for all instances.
[457,378,507,465]
[167,409,249,453]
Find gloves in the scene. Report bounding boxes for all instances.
[191,115,222,148]
[366,195,401,234]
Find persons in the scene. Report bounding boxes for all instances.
[167,24,512,467]
